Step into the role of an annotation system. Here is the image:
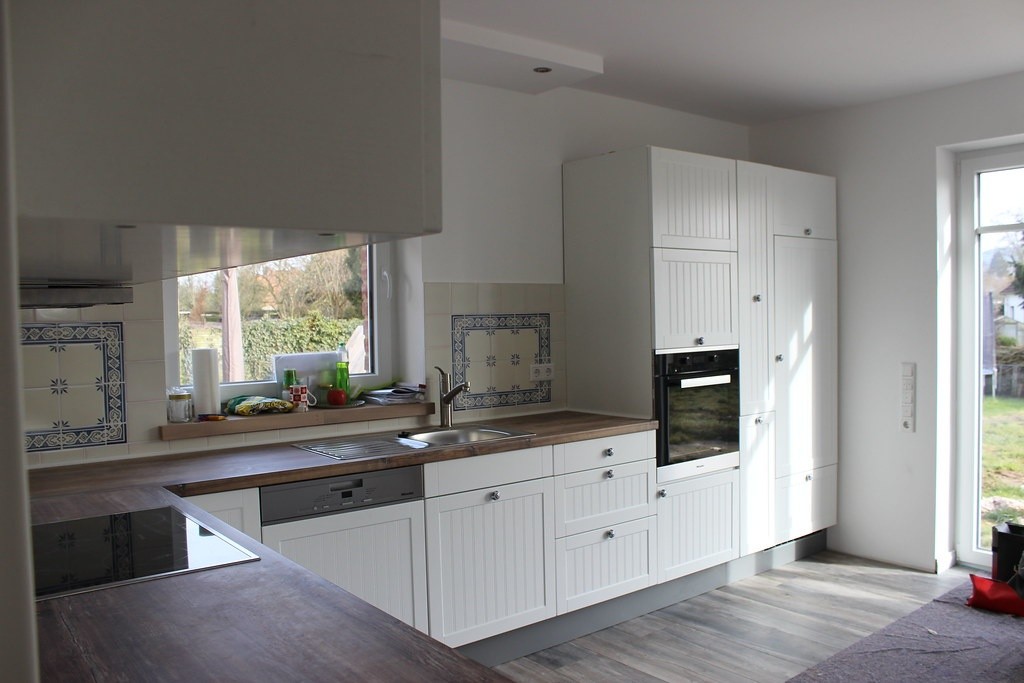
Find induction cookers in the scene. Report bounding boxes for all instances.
[32,505,260,602]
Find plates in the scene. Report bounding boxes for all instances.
[320,401,366,409]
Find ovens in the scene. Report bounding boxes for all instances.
[657,346,741,464]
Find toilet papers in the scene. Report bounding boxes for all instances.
[191,349,220,415]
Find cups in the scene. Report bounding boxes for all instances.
[284,368,296,390]
[282,391,290,401]
[169,394,191,422]
[318,384,333,403]
[289,385,316,413]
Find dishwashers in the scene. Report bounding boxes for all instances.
[259,465,430,637]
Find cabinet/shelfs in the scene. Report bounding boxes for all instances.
[261,500,430,638]
[181,487,262,546]
[562,145,741,422]
[654,468,739,610]
[554,429,659,646]
[737,158,838,580]
[425,444,557,668]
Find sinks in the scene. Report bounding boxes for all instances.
[398,422,538,447]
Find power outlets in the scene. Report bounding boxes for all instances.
[900,377,916,432]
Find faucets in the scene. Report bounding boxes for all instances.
[433,365,472,427]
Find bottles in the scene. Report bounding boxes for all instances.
[337,343,349,389]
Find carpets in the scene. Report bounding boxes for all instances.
[786,576,1024,683]
